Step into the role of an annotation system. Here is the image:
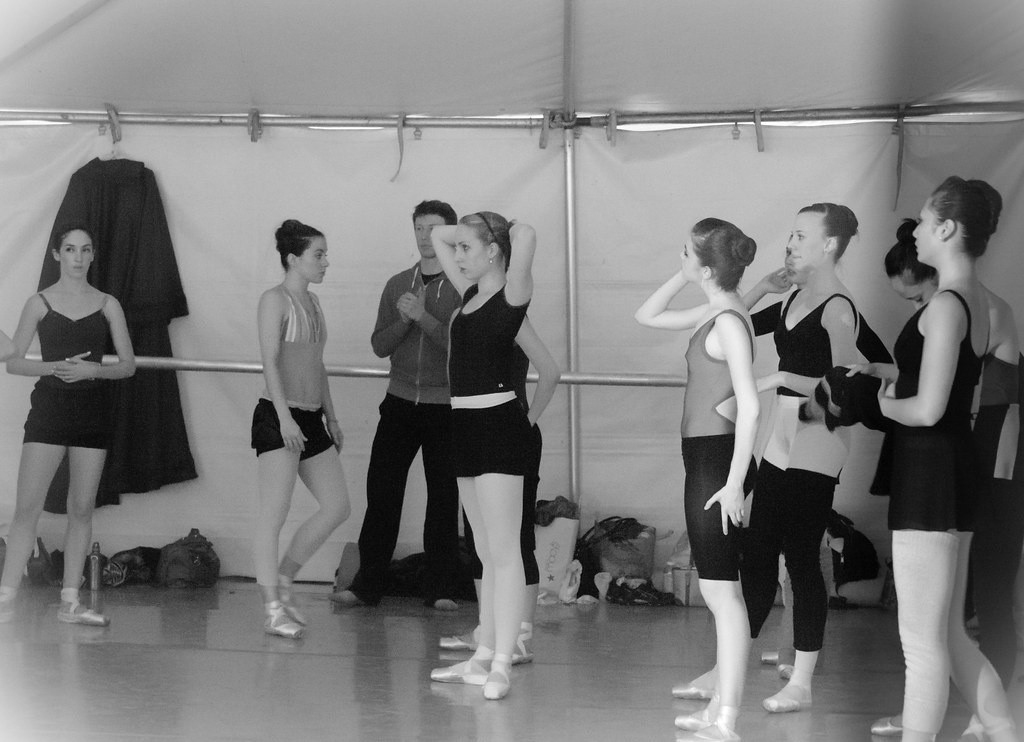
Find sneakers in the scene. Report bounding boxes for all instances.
[605,575,675,606]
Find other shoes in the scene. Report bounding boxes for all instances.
[870,717,903,736]
[672,672,714,699]
[674,709,740,742]
[57,600,110,626]
[264,600,307,638]
[0,611,16,624]
[778,663,795,679]
[431,657,489,685]
[483,667,510,699]
[511,652,534,665]
[762,693,812,712]
[956,714,987,742]
[439,626,482,650]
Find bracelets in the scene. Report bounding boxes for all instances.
[88,366,99,380]
[326,419,338,423]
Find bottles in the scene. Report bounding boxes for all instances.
[87,541,103,591]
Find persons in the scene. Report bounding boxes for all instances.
[635,176,1024,742]
[0,221,136,625]
[329,199,562,700]
[251,220,352,638]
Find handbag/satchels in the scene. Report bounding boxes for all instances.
[0,528,220,588]
[386,495,886,607]
